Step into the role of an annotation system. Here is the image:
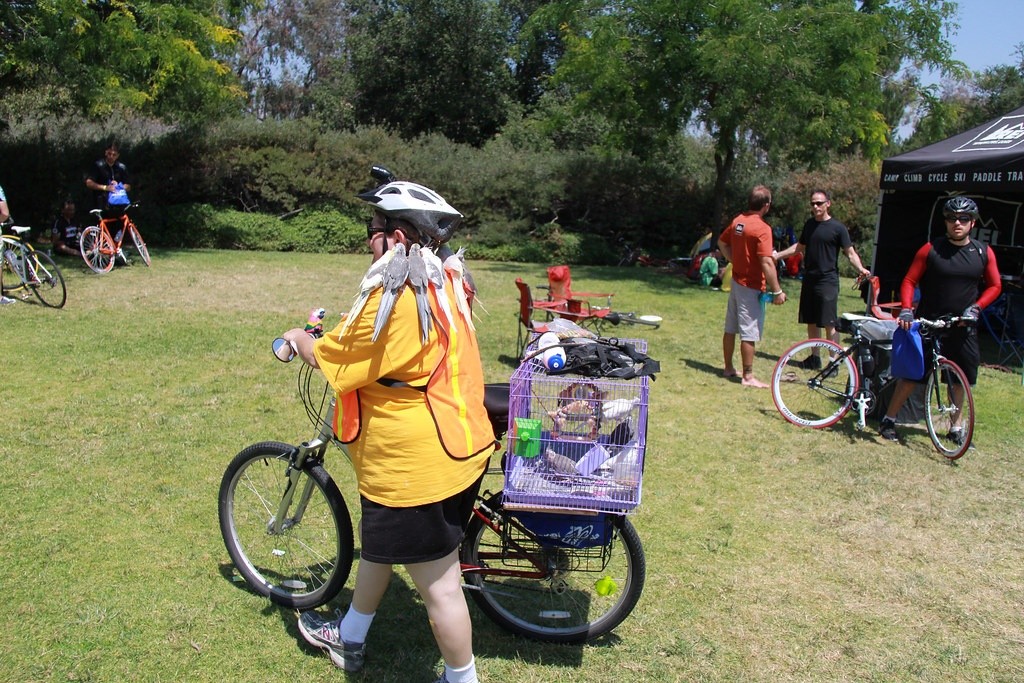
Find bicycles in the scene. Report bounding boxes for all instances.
[79,203,151,274]
[0,217,66,309]
[216,333,645,644]
[770,313,975,460]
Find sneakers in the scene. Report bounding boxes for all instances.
[879,418,898,441]
[432,666,450,683]
[0,296,16,304]
[946,429,976,449]
[298,609,366,672]
[821,362,838,377]
[797,355,821,370]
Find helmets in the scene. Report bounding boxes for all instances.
[943,197,979,216]
[359,181,464,243]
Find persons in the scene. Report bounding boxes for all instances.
[719,184,788,388]
[772,188,871,379]
[0,144,130,304]
[879,198,1001,450]
[693,244,806,292]
[286,180,496,683]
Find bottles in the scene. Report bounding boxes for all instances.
[538,333,567,371]
[4,248,17,267]
[858,348,875,377]
[114,230,122,242]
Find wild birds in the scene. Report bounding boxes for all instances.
[601,396,639,447]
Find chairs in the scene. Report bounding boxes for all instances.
[516,266,615,363]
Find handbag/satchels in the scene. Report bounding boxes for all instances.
[108,179,130,205]
[891,323,924,379]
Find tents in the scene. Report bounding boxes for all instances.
[873,105,1024,370]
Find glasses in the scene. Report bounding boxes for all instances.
[367,223,393,240]
[944,216,972,223]
[810,201,828,206]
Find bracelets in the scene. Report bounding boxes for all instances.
[104,185,107,191]
[770,289,783,295]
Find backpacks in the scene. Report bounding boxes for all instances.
[545,337,660,380]
[686,256,715,281]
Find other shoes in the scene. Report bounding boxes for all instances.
[709,286,719,291]
